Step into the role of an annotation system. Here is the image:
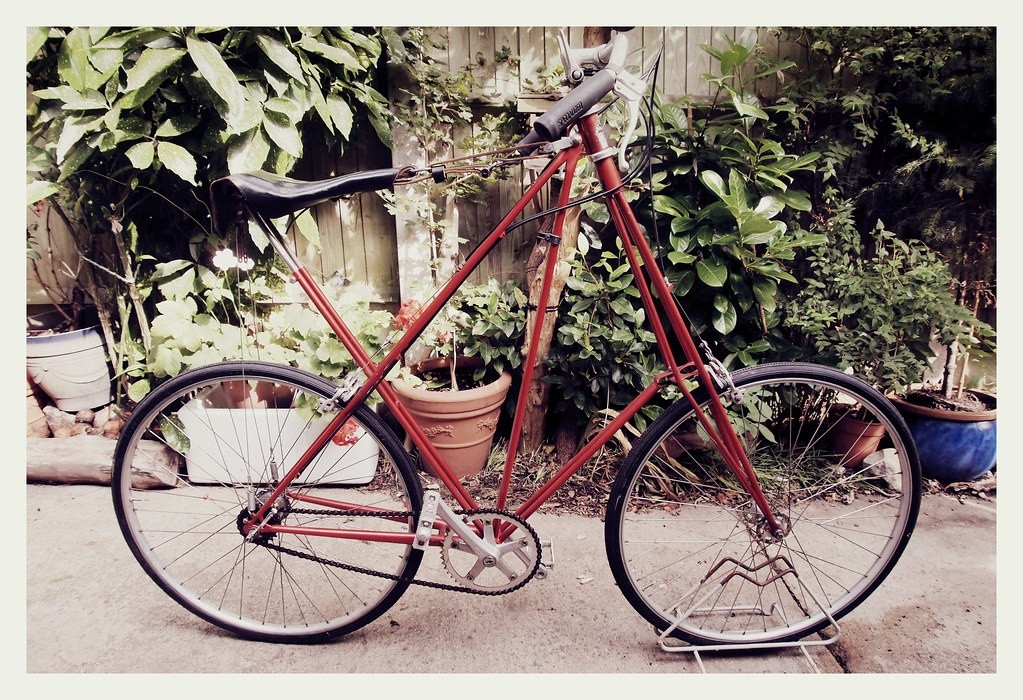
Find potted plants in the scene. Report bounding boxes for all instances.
[26,200,117,412]
[798,216,963,465]
[389,174,513,480]
[883,41,998,481]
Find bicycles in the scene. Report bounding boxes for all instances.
[112,32,924,655]
